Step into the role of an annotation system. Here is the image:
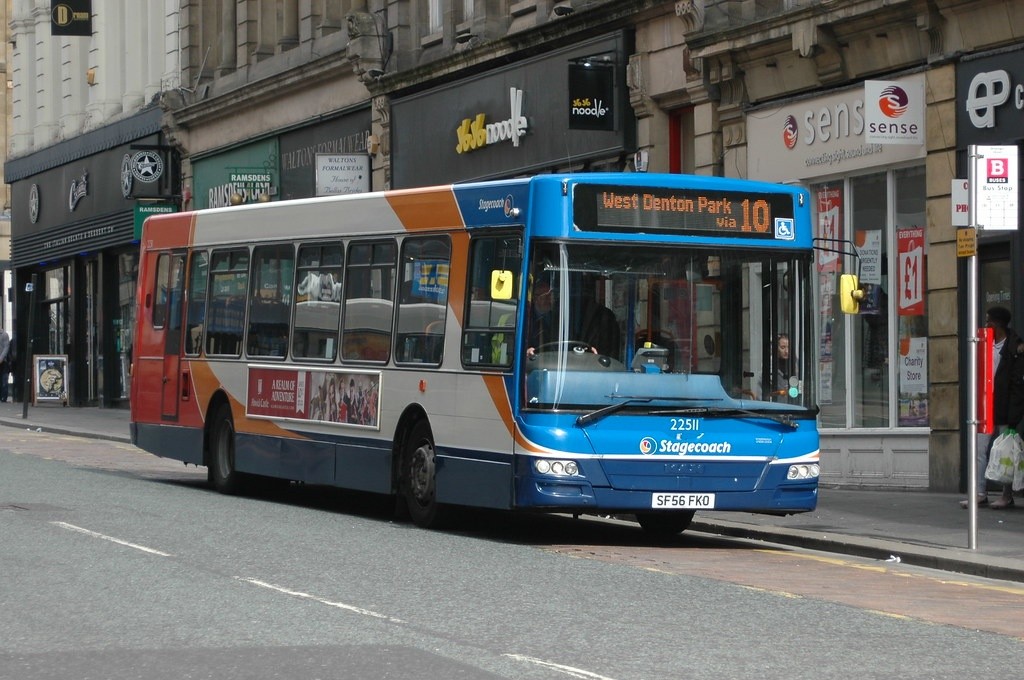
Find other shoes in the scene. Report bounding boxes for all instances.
[990,497,1014,509]
[961,494,989,508]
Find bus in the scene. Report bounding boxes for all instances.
[127,171,863,535]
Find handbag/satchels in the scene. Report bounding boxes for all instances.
[984,431,1024,493]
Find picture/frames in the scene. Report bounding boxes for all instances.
[33,354,69,407]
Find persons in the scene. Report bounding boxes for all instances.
[0,328,11,402]
[297,258,338,302]
[756,333,793,406]
[958,306,1024,508]
